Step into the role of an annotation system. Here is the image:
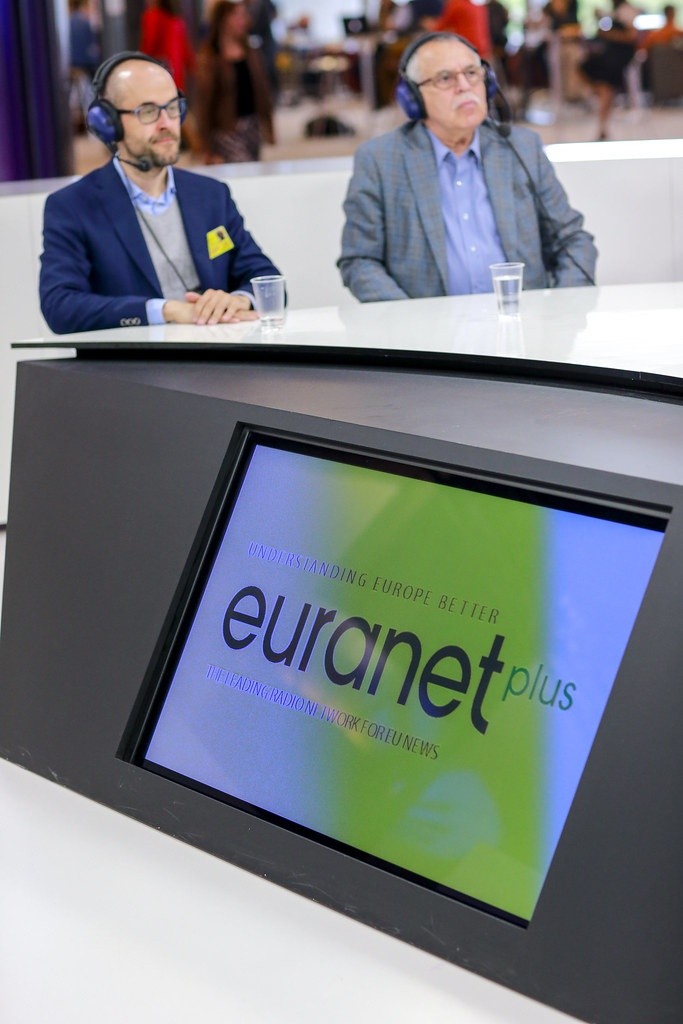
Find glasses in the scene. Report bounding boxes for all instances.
[118,97,186,125]
[417,66,484,90]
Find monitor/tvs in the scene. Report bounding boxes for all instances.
[127,431,674,930]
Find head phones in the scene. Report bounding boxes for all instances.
[396,31,499,120]
[85,51,188,143]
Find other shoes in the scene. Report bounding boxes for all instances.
[596,132,610,142]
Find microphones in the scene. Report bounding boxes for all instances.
[106,141,154,172]
[497,87,512,138]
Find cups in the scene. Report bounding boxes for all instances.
[490,263,526,317]
[250,276,285,330]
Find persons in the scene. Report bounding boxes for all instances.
[372,0,509,81]
[39,59,286,334]
[335,36,598,303]
[520,0,683,143]
[67,0,281,162]
